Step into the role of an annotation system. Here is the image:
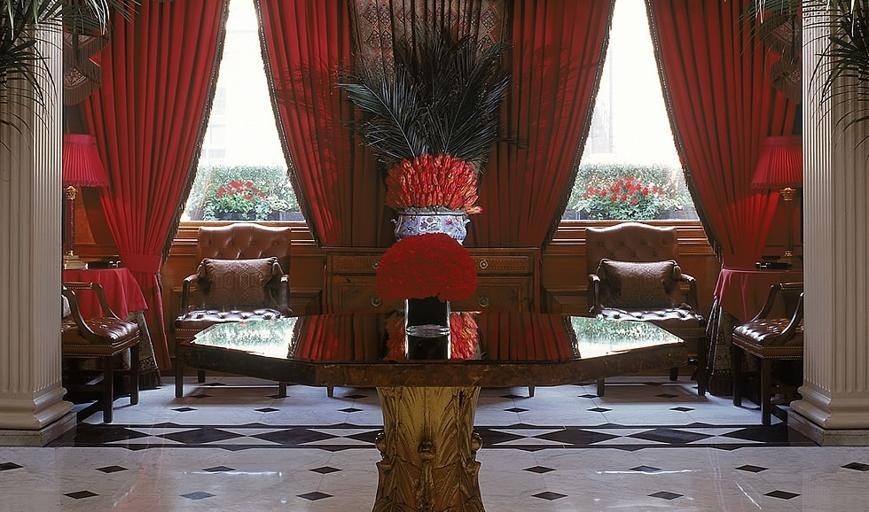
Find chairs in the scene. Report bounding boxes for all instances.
[61,280,141,424]
[585,222,699,397]
[730,280,803,426]
[175,222,293,398]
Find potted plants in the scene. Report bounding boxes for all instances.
[335,16,510,245]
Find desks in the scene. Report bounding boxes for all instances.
[701,268,803,396]
[178,308,688,512]
[63,267,164,388]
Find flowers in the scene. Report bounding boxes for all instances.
[383,153,482,215]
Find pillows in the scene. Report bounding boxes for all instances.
[597,258,682,308]
[195,257,284,310]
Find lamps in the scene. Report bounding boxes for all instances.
[752,135,802,271]
[62,132,109,269]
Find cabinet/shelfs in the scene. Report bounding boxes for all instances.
[323,247,542,398]
